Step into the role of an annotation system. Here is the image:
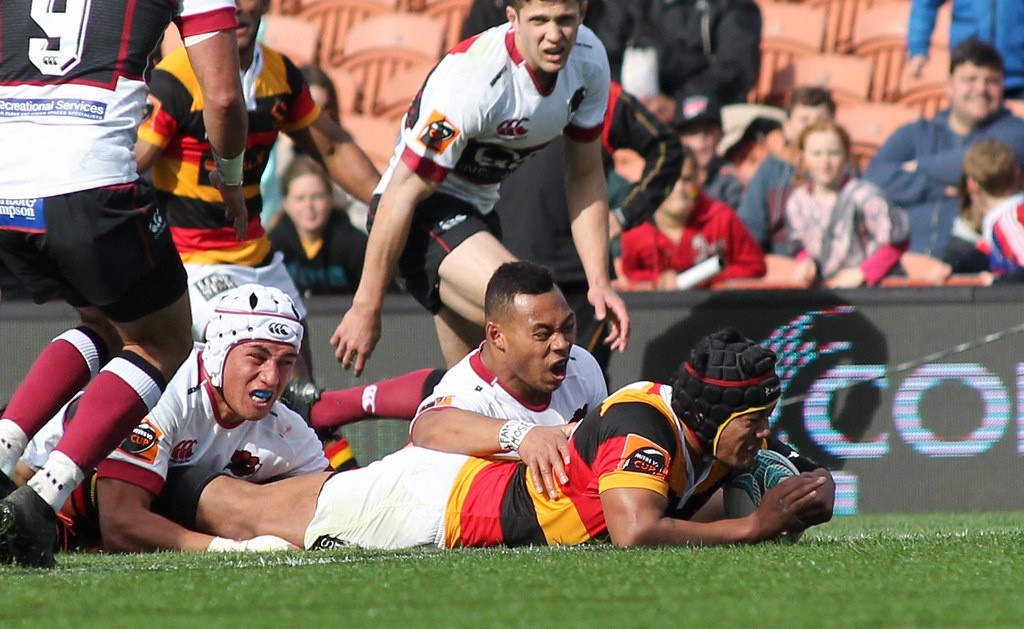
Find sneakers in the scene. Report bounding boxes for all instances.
[0,470,74,571]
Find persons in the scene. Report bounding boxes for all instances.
[58,325,834,550]
[281,0,631,440]
[0,0,247,566]
[409,260,606,498]
[134,0,385,473]
[572,82,683,370]
[0,284,331,553]
[261,66,399,296]
[461,0,1024,288]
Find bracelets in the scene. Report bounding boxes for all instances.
[499,420,536,452]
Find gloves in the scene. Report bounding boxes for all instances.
[206,534,304,554]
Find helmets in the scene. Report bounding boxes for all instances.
[201,283,304,388]
[671,327,782,459]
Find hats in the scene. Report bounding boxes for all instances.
[671,92,721,128]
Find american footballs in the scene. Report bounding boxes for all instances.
[721,448,807,546]
